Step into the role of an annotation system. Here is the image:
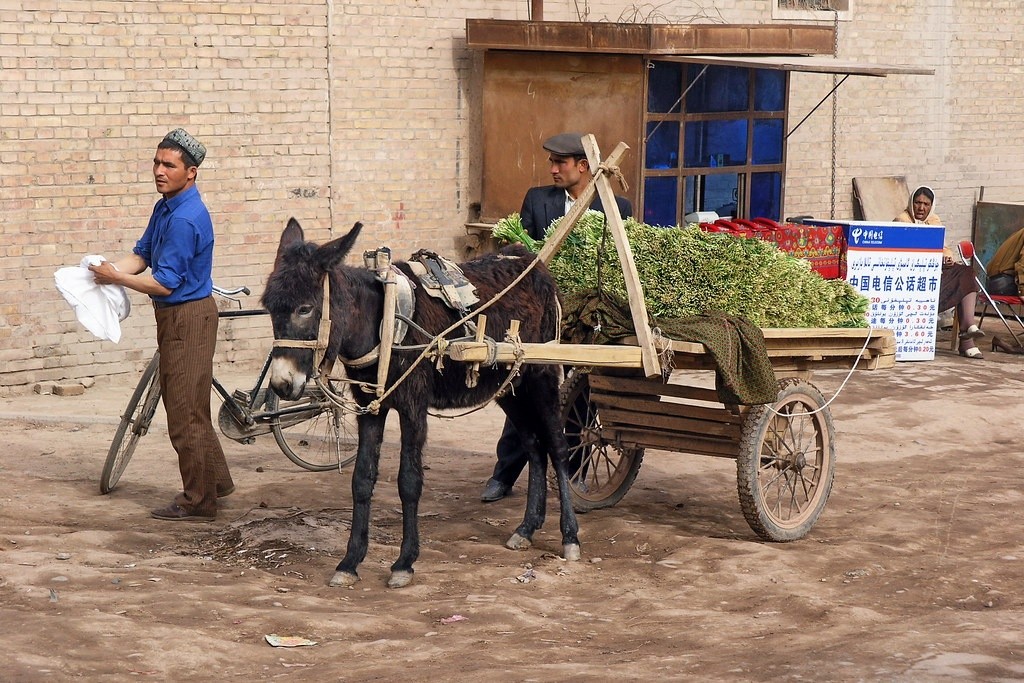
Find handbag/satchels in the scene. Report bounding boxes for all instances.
[984,268,1018,296]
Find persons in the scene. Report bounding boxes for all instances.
[481,133,633,503]
[88,127,236,522]
[892,186,985,359]
[986,228,1024,301]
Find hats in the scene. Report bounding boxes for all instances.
[163,127,207,167]
[543,133,585,156]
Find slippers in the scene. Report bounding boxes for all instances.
[958,324,985,340]
[959,347,985,359]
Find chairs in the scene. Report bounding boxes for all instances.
[957,241,1024,349]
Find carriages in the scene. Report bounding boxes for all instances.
[256,132,900,591]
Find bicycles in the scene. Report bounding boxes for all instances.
[97,280,364,496]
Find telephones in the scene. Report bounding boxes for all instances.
[698,217,789,231]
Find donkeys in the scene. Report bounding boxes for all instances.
[258,217,580,591]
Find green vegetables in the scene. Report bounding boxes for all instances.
[490,209,870,329]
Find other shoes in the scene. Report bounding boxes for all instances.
[175,485,235,501]
[573,482,589,494]
[150,501,216,522]
[480,477,512,501]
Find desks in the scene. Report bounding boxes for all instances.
[697,218,945,361]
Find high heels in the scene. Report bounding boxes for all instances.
[991,336,1019,354]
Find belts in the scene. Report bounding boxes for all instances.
[152,299,201,309]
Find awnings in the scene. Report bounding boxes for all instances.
[644,54,936,77]
